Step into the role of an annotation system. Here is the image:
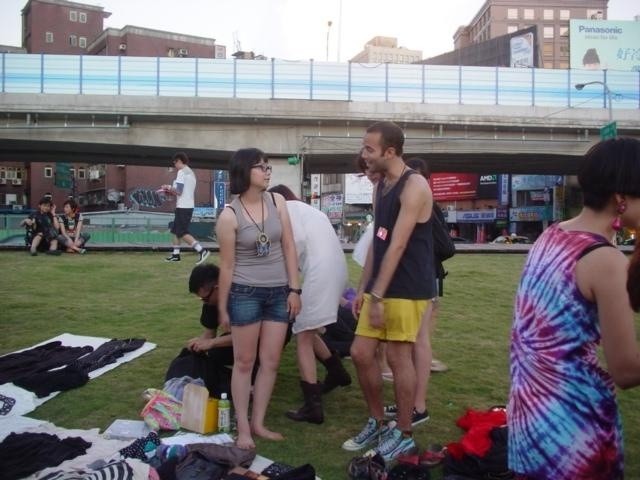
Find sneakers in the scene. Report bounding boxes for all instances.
[342,406,429,462]
[196,248,210,265]
[164,255,181,263]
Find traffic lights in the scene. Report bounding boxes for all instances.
[288,155,299,167]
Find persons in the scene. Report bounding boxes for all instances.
[267,184,352,425]
[19,197,61,257]
[185,260,290,401]
[219,148,301,447]
[403,155,450,373]
[55,200,92,255]
[582,48,601,69]
[159,150,210,264]
[344,121,432,463]
[508,139,639,480]
[356,145,429,427]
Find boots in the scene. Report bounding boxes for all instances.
[286,379,322,425]
[323,354,351,395]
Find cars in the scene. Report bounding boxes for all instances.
[340,239,349,244]
[450,236,473,245]
[488,233,530,245]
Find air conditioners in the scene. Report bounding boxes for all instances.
[0,177,7,185]
[11,178,22,186]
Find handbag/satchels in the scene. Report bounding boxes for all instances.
[430,199,456,262]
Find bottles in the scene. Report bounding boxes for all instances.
[218,393,232,431]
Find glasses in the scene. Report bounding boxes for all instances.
[200,289,215,303]
[252,163,272,172]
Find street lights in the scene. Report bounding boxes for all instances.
[325,20,336,59]
[576,78,614,128]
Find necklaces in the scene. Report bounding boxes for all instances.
[238,192,270,246]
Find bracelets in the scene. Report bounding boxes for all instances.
[370,291,383,303]
[52,215,58,217]
